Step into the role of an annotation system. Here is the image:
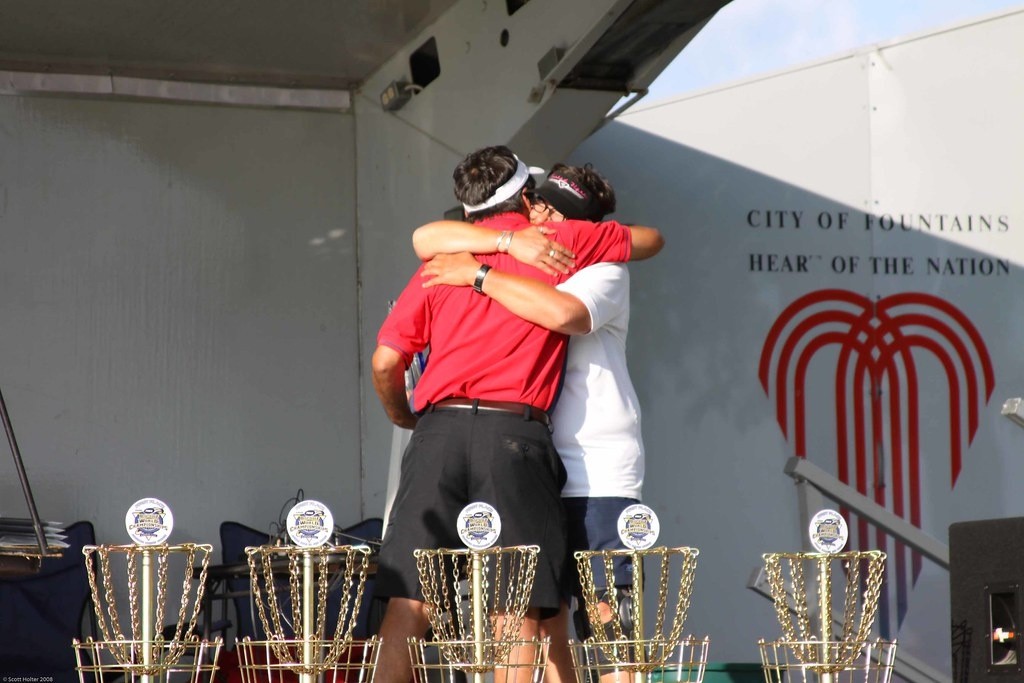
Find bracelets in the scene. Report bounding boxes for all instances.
[473,264,492,293]
[496,230,506,251]
[504,232,514,254]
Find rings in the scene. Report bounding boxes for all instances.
[549,250,555,257]
[540,226,543,234]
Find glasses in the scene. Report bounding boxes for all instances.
[533,197,565,223]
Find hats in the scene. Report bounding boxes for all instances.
[461,153,544,214]
[522,169,602,221]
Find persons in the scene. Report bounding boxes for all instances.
[371,145,666,683]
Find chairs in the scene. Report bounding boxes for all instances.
[0,521,174,683]
[220,515,387,670]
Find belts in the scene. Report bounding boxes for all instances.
[434,393,555,432]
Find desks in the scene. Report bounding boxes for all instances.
[188,552,484,683]
